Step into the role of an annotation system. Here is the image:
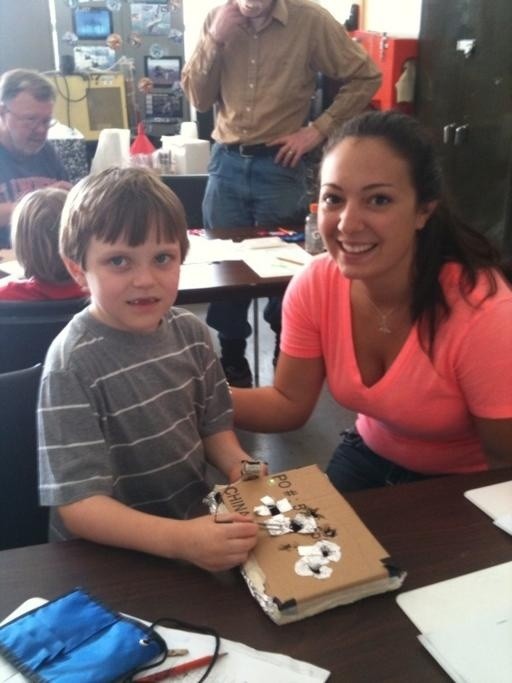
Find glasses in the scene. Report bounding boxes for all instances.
[4,109,57,127]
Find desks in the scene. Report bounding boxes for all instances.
[1,223,327,389]
[0,466,512,683]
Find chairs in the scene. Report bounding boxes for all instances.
[1,293,91,375]
[0,359,49,549]
[158,172,211,228]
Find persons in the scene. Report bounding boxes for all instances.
[0,68,74,250]
[34,166,268,575]
[178,0,380,387]
[0,187,90,302]
[228,110,512,491]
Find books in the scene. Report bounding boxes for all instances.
[204,464,406,627]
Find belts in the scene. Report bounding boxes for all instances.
[227,143,279,158]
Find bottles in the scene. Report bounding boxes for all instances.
[303,203,325,254]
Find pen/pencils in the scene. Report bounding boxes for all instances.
[249,245,283,249]
[133,652,228,683]
[277,257,304,265]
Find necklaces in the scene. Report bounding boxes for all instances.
[363,295,397,335]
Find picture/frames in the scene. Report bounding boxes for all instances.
[70,6,112,40]
[143,53,181,88]
